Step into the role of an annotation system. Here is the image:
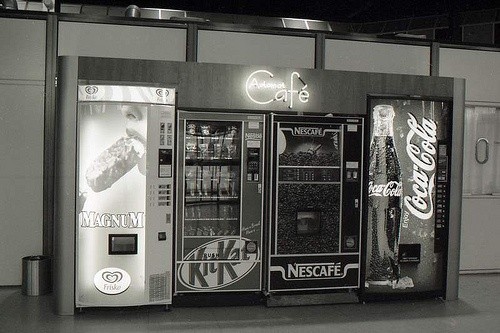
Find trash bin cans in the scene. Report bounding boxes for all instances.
[22,255,50,297]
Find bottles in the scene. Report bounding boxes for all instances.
[365,104,404,288]
